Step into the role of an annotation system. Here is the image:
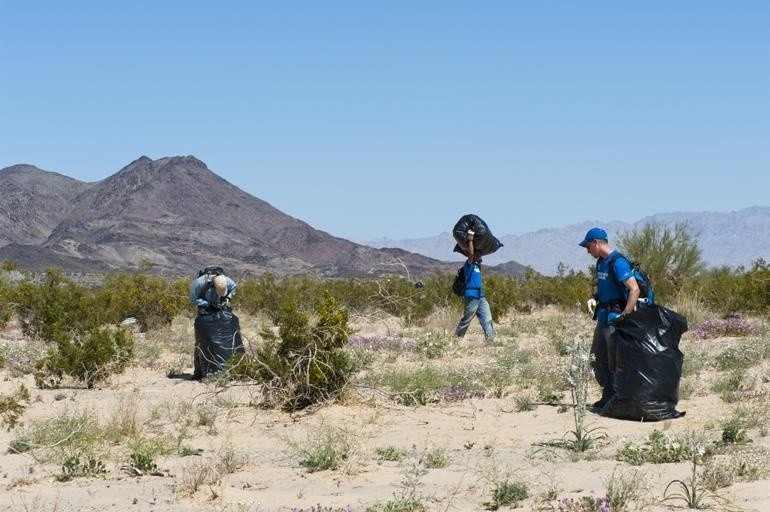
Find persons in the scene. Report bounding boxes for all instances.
[188,274,237,317]
[579,227,640,408]
[455,230,493,343]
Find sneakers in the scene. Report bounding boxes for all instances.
[593,388,615,408]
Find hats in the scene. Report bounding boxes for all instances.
[578,228,608,247]
[214,274,227,296]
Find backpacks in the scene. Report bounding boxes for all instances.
[609,253,654,312]
[199,265,224,299]
[452,263,474,297]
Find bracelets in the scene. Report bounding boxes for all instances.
[468,239,474,241]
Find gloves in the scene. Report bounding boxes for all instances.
[467,229,475,241]
[587,298,597,319]
